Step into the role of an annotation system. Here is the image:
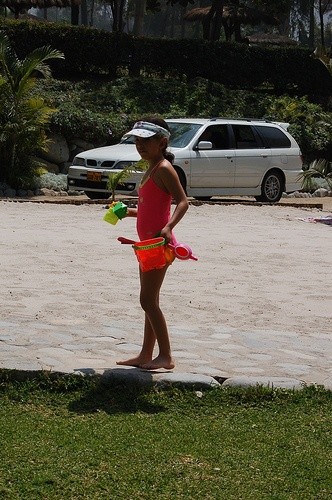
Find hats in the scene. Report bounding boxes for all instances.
[123,121,171,139]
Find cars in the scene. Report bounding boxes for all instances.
[66,117,305,205]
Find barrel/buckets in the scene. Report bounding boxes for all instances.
[132,237,167,272]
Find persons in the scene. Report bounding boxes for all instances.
[109,117,189,369]
